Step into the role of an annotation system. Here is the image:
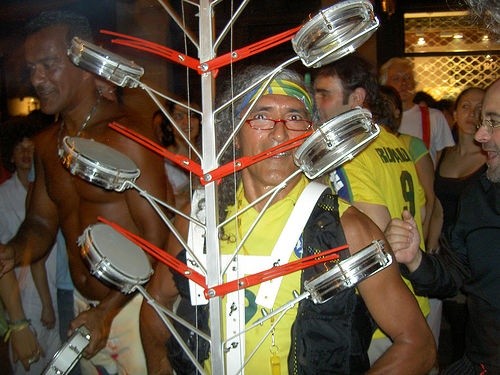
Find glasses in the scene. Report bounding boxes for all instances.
[474,119,500,129]
[175,109,197,120]
[237,116,314,131]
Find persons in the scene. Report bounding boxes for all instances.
[140,65,437,375]
[310,55,500,375]
[0,11,202,375]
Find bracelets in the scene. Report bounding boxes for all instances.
[5,318,37,343]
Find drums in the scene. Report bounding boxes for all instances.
[67,37,145,89]
[304,238,392,304]
[58,136,140,192]
[42,332,90,375]
[76,223,154,294]
[294,106,380,180]
[291,0,379,68]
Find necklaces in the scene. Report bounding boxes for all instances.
[56,90,101,150]
